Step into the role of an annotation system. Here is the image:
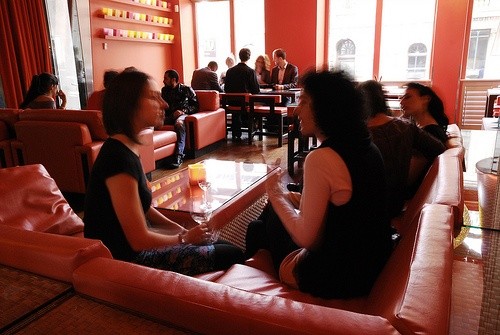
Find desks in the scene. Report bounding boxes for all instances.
[476,159,500,231]
[256,90,302,104]
[484,90,500,119]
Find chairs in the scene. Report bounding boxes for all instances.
[249,91,290,147]
[286,104,317,174]
[219,93,260,144]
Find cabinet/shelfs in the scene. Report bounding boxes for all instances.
[100,0,176,44]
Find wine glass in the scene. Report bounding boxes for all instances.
[190,205,214,251]
[198,175,212,211]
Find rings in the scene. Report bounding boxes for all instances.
[205,232,208,237]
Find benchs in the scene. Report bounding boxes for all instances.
[0,89,464,335]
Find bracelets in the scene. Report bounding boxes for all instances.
[179,233,185,244]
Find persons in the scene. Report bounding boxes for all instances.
[254,55,270,84]
[224,48,259,137]
[285,80,448,209]
[287,82,449,192]
[191,61,224,93]
[85,71,120,112]
[19,73,66,110]
[161,70,197,168]
[82,71,245,276]
[219,57,235,85]
[245,68,394,299]
[266,49,298,134]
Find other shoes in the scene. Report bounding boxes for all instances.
[169,155,183,168]
[287,182,302,192]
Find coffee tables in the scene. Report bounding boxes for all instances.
[149,158,281,238]
